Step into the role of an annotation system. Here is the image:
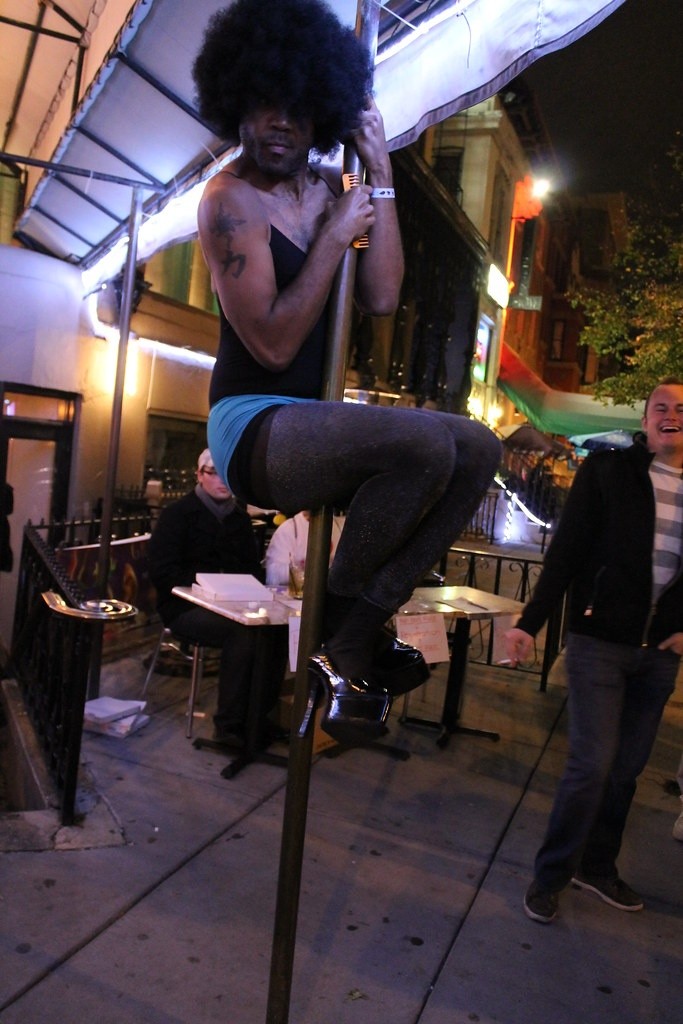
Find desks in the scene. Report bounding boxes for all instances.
[168,584,531,777]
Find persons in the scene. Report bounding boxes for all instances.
[147,448,290,757]
[190,0,503,748]
[507,379,683,923]
[265,507,348,595]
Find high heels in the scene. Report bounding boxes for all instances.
[297,591,398,748]
[323,586,431,698]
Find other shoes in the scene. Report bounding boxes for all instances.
[262,720,292,743]
[220,728,258,755]
[672,810,683,840]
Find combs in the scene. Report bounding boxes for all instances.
[343,173,369,248]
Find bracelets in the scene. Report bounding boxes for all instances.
[371,188,395,198]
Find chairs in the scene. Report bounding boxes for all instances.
[135,585,220,737]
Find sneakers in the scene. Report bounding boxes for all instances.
[522,879,559,922]
[570,874,643,911]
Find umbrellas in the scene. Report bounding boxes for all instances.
[568,428,634,451]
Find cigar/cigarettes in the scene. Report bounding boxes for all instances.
[498,658,518,664]
